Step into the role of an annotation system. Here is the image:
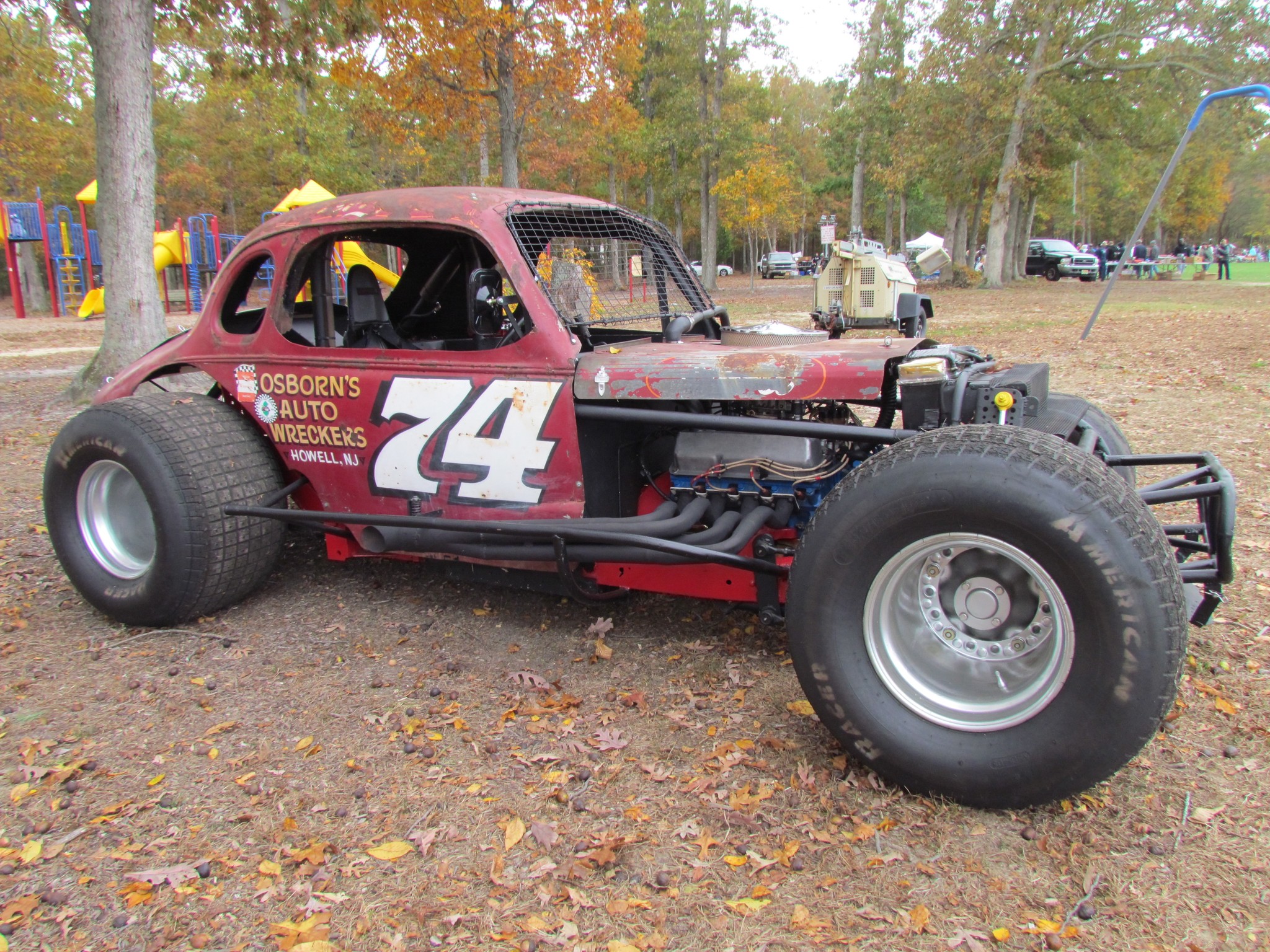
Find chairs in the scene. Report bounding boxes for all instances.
[345,265,424,352]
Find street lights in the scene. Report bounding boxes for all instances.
[817,215,838,257]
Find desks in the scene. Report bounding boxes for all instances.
[162,289,204,306]
[798,265,811,271]
[1105,260,1210,280]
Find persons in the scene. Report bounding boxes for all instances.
[976,244,987,261]
[1076,238,1270,282]
[813,253,824,263]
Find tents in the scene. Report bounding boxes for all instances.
[905,232,945,249]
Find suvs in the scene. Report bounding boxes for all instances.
[761,252,798,279]
[1024,239,1100,282]
[42,190,1244,815]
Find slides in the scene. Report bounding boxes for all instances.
[78,245,175,318]
[343,241,403,288]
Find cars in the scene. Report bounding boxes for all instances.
[685,261,733,277]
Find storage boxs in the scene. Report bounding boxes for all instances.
[1164,257,1178,263]
[1161,272,1182,280]
[789,275,801,280]
[1185,255,1203,263]
[1108,271,1136,280]
[1193,270,1218,280]
[784,272,790,279]
